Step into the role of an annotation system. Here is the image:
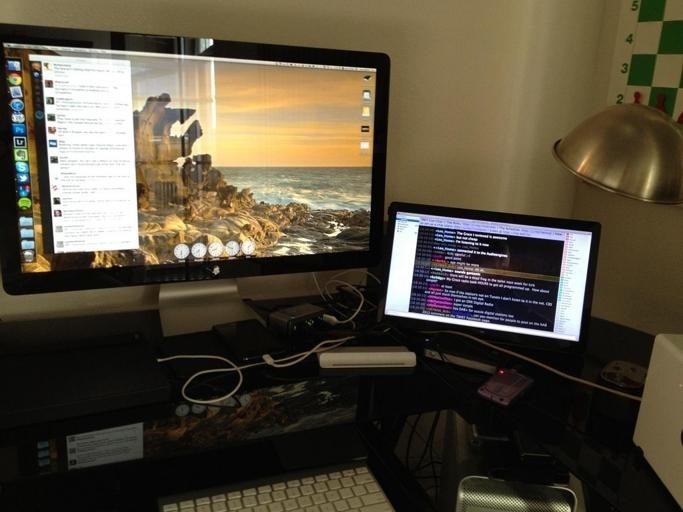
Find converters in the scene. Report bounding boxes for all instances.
[269,302,325,338]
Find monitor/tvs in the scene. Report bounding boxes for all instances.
[0,25,392,337]
[377,201,601,381]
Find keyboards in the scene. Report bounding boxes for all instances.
[156,459,397,512]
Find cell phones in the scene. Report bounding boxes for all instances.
[213,318,285,361]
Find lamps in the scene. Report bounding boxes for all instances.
[551,100,682,204]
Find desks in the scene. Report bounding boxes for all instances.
[0,313,683,512]
